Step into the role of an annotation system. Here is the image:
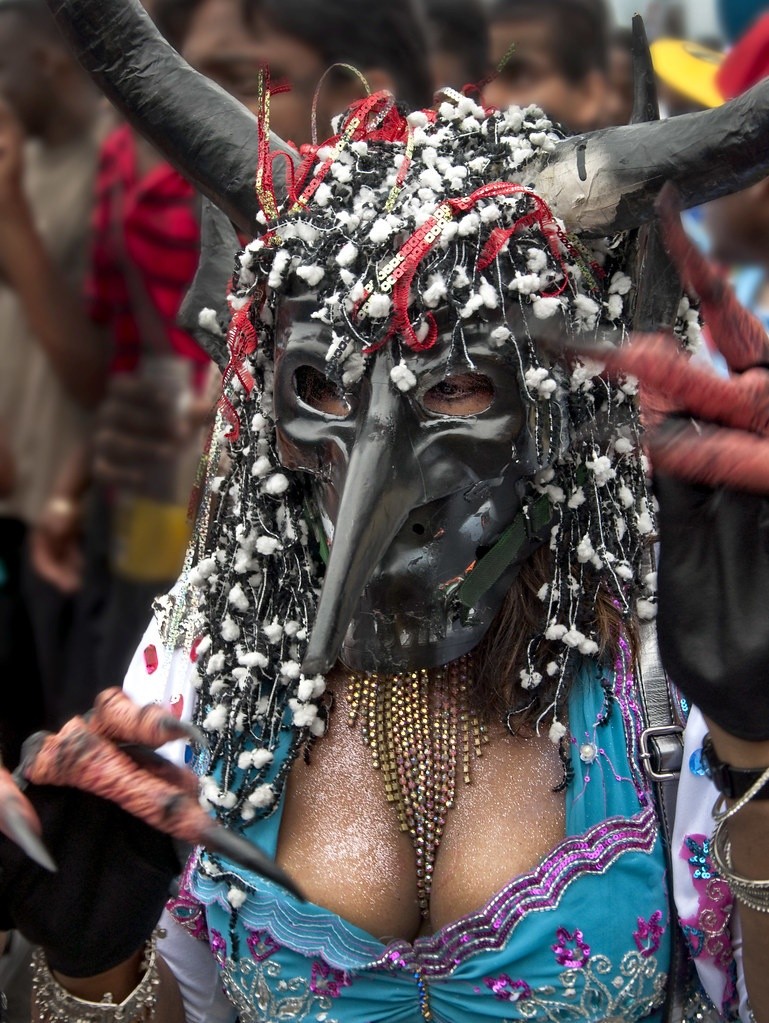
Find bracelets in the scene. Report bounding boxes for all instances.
[708,770,769,914]
[31,923,167,1023]
[703,733,769,802]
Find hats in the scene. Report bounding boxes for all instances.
[649,14,769,108]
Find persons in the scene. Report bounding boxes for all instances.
[1,0,768,683]
[1,97,769,1022]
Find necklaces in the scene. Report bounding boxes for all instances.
[346,664,491,919]
